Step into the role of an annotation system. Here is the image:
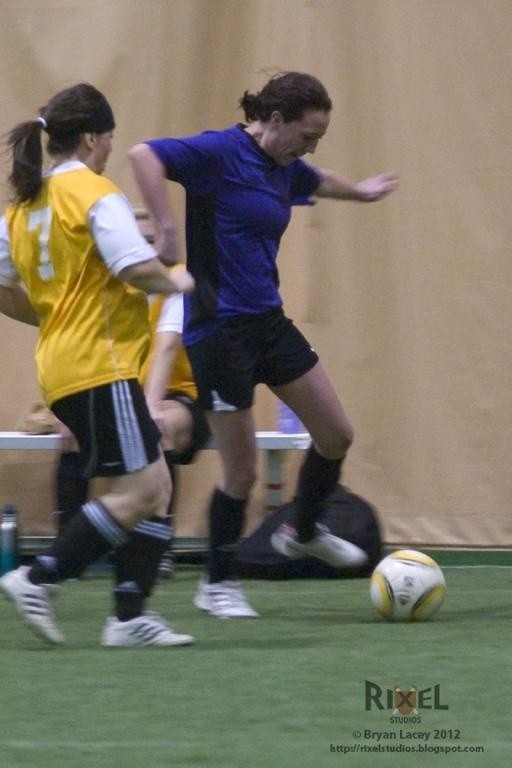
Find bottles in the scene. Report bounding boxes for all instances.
[0,503,18,578]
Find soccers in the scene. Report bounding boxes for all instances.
[370,550,446,622]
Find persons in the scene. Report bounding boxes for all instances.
[53,206,210,583]
[124,71,400,621]
[0,84,197,650]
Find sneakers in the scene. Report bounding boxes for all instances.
[189,573,260,619]
[0,564,68,646]
[271,520,369,570]
[100,612,198,649]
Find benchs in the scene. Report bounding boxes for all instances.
[0,427,314,524]
